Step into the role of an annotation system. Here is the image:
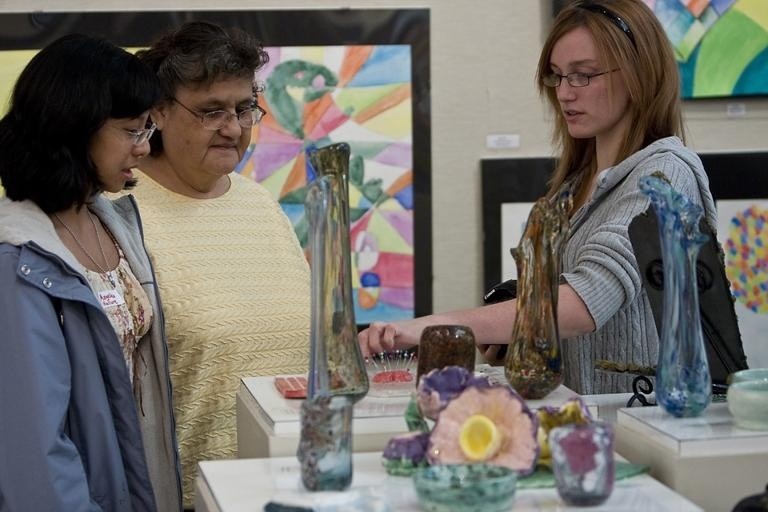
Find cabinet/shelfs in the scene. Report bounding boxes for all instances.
[195,451,706,512]
[235,366,601,459]
[614,402,768,512]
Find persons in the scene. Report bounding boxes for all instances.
[101,23,310,511]
[0,34,184,510]
[357,0,748,397]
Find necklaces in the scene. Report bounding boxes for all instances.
[54,210,116,288]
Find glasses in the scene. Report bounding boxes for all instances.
[170,93,266,129]
[106,120,159,146]
[541,60,621,89]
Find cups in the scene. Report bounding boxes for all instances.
[299,398,354,492]
[548,423,614,506]
[724,367,768,428]
[412,463,520,512]
